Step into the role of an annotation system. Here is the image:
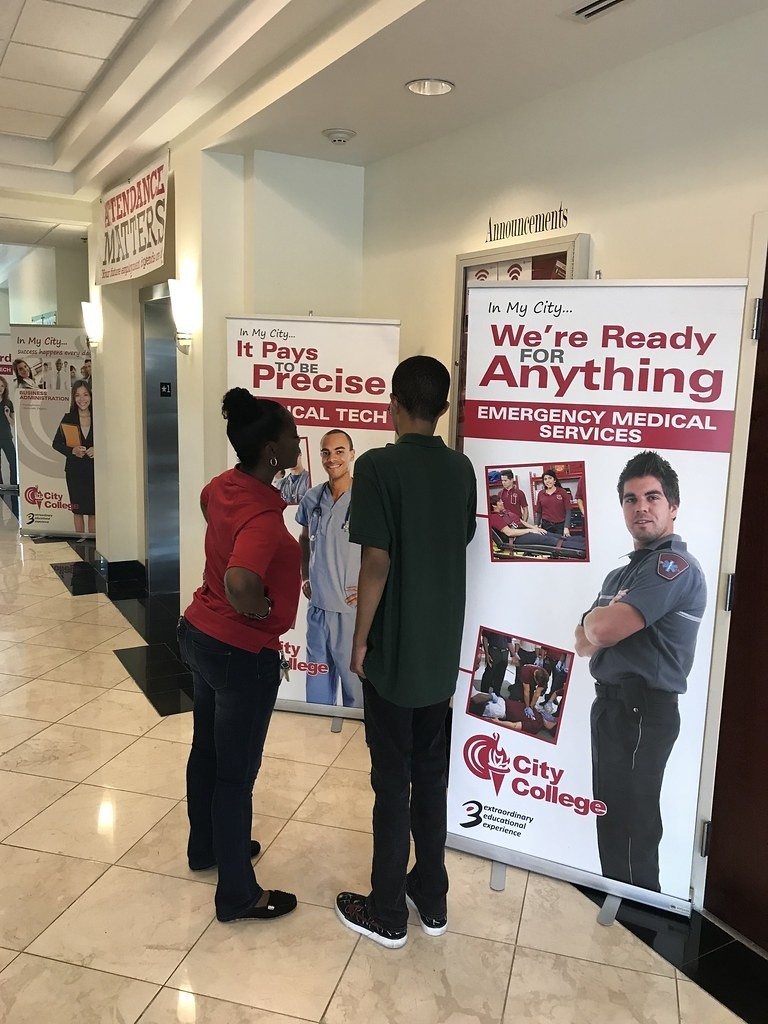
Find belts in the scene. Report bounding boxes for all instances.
[595,682,679,704]
[490,645,509,652]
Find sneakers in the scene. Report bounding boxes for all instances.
[405,885,448,936]
[335,892,407,949]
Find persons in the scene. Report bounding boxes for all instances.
[575,474,585,538]
[12,358,40,390]
[470,628,569,722]
[468,692,557,733]
[294,430,364,711]
[173,387,300,922]
[51,379,96,534]
[0,376,18,486]
[42,358,92,390]
[575,448,709,892]
[271,448,309,504]
[335,354,478,951]
[489,495,586,551]
[497,469,529,522]
[535,471,572,538]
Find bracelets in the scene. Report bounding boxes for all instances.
[254,597,273,619]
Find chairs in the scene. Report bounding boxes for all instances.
[491,527,586,559]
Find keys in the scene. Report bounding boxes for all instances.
[279,660,290,683]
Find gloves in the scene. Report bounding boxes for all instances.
[555,661,562,672]
[524,707,534,716]
[491,693,497,704]
[542,711,555,722]
[564,667,569,673]
[545,701,553,714]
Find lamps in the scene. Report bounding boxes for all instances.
[81,301,102,349]
[168,278,197,355]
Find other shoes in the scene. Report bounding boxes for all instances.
[192,840,261,872]
[237,891,296,919]
